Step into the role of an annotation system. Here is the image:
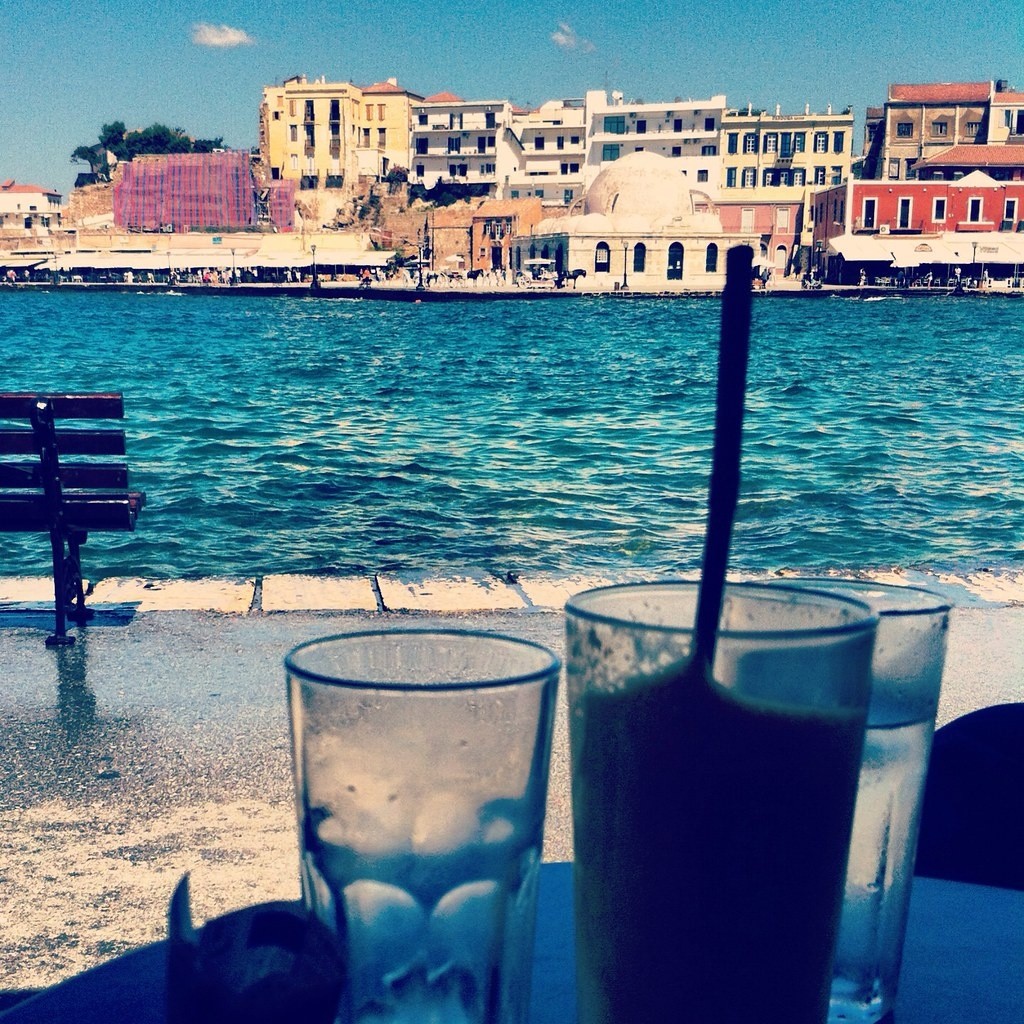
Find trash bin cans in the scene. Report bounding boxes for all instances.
[1015,278,1020,287]
[316,279,321,287]
[615,282,619,291]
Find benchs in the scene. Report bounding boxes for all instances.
[306,274,331,282]
[336,274,356,281]
[0,391,147,642]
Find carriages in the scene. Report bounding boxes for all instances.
[516,258,587,290]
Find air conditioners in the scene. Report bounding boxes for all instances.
[417,108,425,113]
[683,139,691,144]
[664,111,674,117]
[693,139,699,143]
[484,105,492,111]
[693,110,701,116]
[880,224,891,235]
[630,112,636,118]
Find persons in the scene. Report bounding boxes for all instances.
[58,267,71,276]
[358,266,505,289]
[533,267,545,280]
[513,264,521,276]
[954,265,961,279]
[6,270,18,282]
[897,269,904,288]
[859,268,866,286]
[761,269,771,289]
[24,270,30,283]
[137,272,155,283]
[984,268,988,277]
[284,270,301,283]
[168,267,241,286]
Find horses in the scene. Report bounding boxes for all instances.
[557,269,586,289]
[467,268,484,288]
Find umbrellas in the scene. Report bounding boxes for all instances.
[752,256,775,272]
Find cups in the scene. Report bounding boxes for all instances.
[719,577,954,1024]
[281,629,562,1024]
[563,580,882,1024]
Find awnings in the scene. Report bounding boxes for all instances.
[829,233,1024,268]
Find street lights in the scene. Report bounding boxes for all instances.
[415,238,425,288]
[621,241,629,291]
[230,246,236,285]
[165,250,175,284]
[817,238,824,284]
[309,244,316,286]
[970,240,980,288]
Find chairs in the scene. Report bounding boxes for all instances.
[875,275,968,287]
